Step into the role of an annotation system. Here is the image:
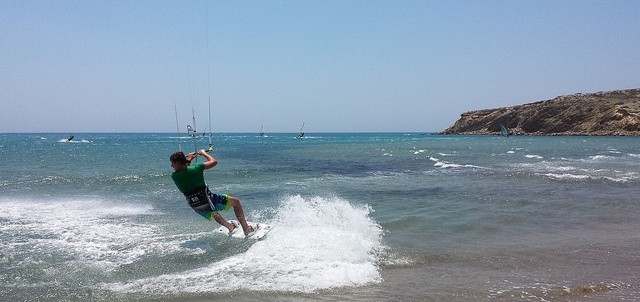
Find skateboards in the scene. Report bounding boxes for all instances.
[217,220,273,239]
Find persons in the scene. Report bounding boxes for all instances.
[169,149,259,236]
